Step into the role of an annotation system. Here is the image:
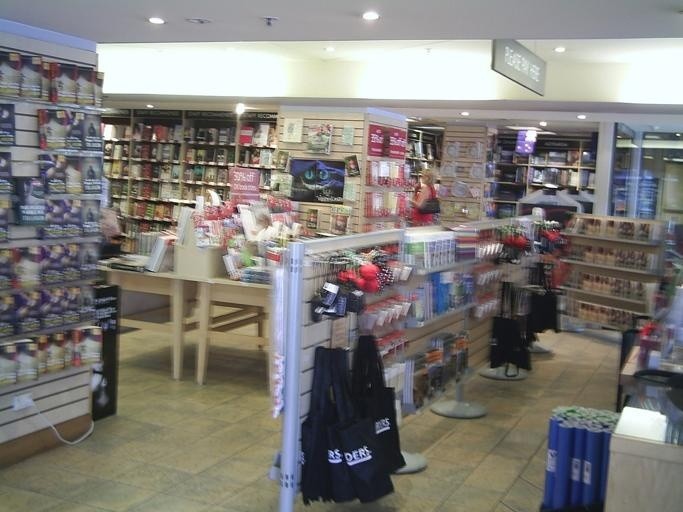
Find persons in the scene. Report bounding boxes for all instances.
[408,168,438,224]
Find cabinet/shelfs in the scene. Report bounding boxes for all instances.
[557,211,665,339]
[100,112,275,243]
[406,129,599,217]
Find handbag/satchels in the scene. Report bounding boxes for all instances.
[487,262,561,372]
[300,332,409,507]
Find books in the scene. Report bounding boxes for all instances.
[101,123,360,257]
[0,49,106,385]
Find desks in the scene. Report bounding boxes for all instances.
[97,251,273,390]
